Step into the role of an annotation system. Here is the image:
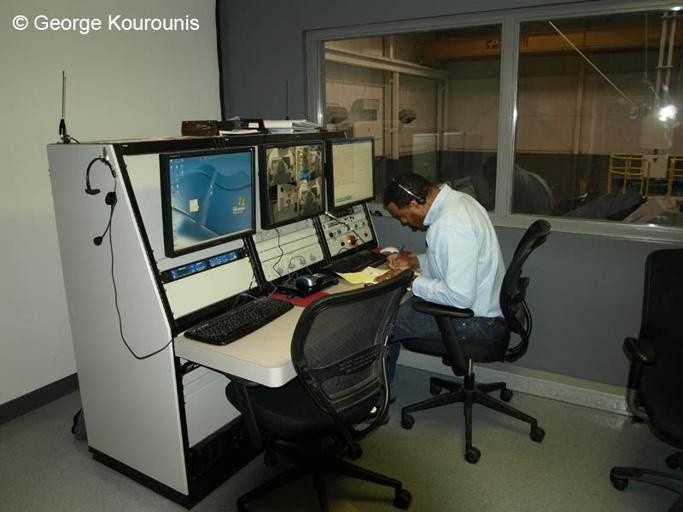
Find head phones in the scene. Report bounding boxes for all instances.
[85,158,116,204]
[392,176,426,205]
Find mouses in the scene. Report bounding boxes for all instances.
[380,246,400,255]
[296,275,323,289]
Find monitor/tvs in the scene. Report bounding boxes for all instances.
[411,132,440,182]
[159,146,256,258]
[258,140,326,230]
[440,131,466,181]
[326,137,377,212]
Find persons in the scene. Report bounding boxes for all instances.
[377,169,510,417]
[485,155,554,214]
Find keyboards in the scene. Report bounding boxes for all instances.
[184,300,294,346]
[323,250,386,274]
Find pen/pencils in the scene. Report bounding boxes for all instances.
[395,244,405,261]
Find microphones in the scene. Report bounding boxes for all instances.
[94,203,115,246]
[411,226,417,232]
[324,210,368,249]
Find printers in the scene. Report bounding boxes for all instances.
[181,118,264,136]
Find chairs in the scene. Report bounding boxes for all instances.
[542,188,592,217]
[226,270,412,512]
[609,246,682,512]
[401,220,554,464]
[607,152,683,197]
